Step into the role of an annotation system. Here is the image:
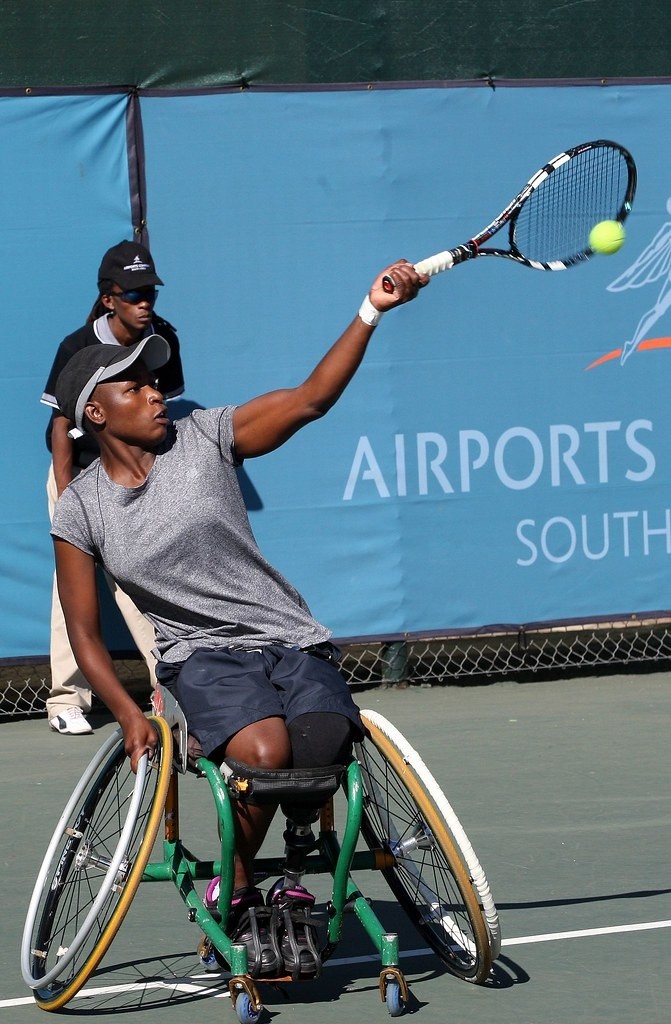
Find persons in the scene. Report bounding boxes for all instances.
[40,240,185,734]
[49,256,431,980]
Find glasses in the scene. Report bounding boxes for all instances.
[109,290,159,304]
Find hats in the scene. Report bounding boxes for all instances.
[98,239,164,290]
[55,334,172,434]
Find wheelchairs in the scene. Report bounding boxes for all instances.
[20,681,500,1024]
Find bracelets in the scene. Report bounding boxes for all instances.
[358,295,384,327]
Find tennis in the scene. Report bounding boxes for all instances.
[589,221,626,256]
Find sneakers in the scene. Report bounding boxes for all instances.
[266,877,320,973]
[205,876,280,975]
[49,706,93,735]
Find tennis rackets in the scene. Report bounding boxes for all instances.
[382,138,638,293]
